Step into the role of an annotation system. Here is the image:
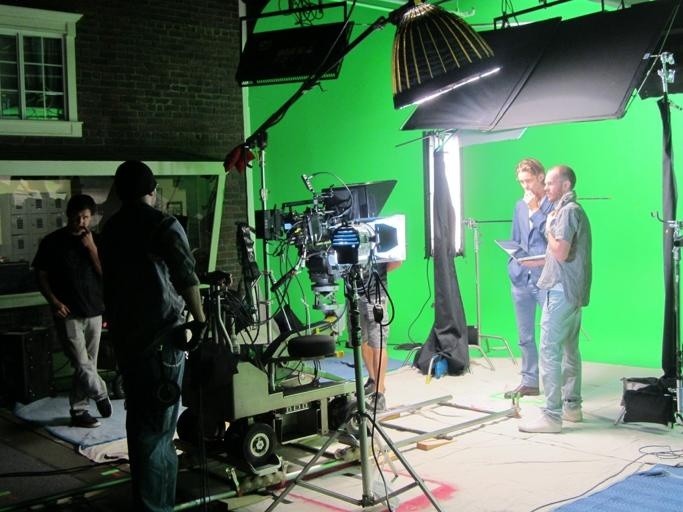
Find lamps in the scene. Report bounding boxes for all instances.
[300,0,504,110]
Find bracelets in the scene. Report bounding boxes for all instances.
[543,230,550,239]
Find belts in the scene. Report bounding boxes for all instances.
[527,272,531,281]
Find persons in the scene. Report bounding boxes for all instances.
[357,257,402,413]
[29,193,112,430]
[515,165,594,434]
[503,158,555,401]
[96,160,207,511]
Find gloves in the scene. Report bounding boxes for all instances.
[182,319,208,353]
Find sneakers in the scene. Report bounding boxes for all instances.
[368,390,387,413]
[94,396,111,418]
[517,411,562,434]
[560,405,584,423]
[70,409,98,426]
[354,376,376,397]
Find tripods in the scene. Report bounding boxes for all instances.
[265,268,443,512]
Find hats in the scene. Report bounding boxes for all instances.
[108,158,157,203]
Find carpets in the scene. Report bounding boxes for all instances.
[550,465,683,512]
[0,349,409,449]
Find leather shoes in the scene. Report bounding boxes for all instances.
[502,382,540,399]
